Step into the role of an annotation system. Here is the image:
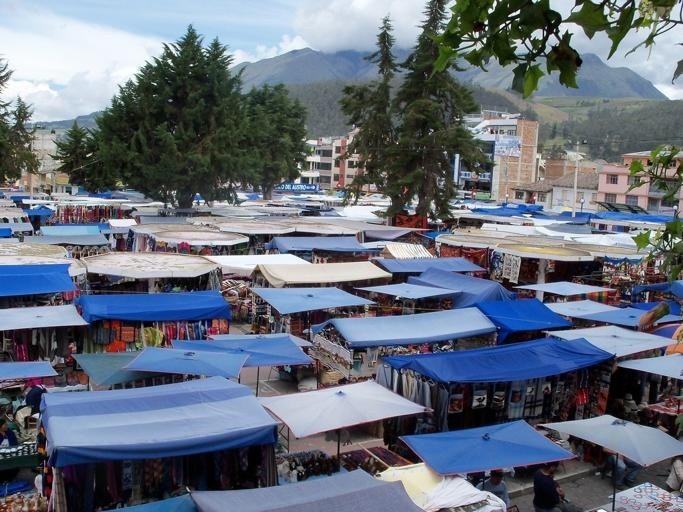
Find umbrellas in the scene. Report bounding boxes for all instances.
[396,419,577,492]
[257,379,434,472]
[353,282,461,302]
[247,286,377,339]
[0,303,89,358]
[535,414,683,512]
[71,331,314,398]
[543,298,683,413]
[0,362,58,379]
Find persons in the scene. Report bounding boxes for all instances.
[14,380,46,430]
[533,461,565,512]
[600,446,643,490]
[35,462,43,497]
[475,468,509,510]
[0,417,18,448]
[666,458,683,493]
